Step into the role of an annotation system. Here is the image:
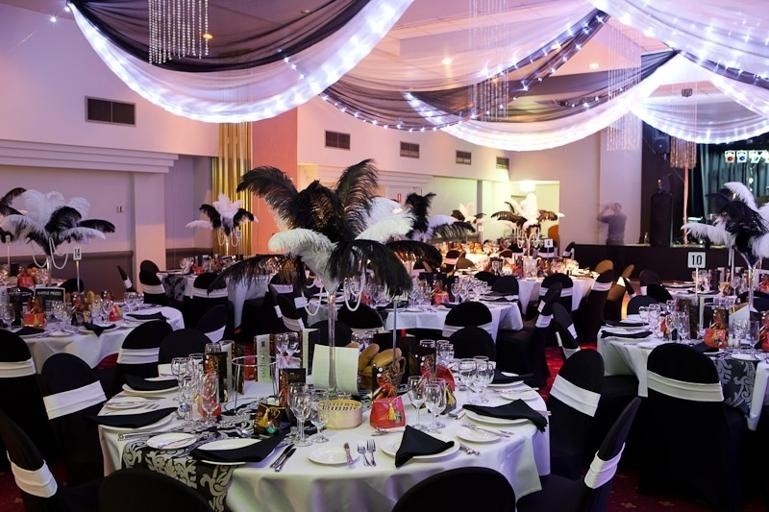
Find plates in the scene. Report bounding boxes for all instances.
[49,329,75,337]
[197,438,275,465]
[12,328,47,338]
[121,377,180,396]
[467,398,528,425]
[78,322,120,333]
[146,431,198,450]
[122,309,169,322]
[498,386,538,402]
[605,319,652,330]
[98,408,174,432]
[377,431,460,460]
[306,441,361,466]
[704,347,724,355]
[611,329,648,343]
[638,341,658,349]
[118,321,140,328]
[731,353,765,361]
[106,395,148,408]
[487,371,524,387]
[457,425,502,442]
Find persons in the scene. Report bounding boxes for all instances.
[596,202,628,246]
[16,264,37,289]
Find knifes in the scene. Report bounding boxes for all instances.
[274,448,296,472]
[344,442,354,467]
[270,443,294,468]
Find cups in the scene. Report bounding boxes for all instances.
[336,391,352,401]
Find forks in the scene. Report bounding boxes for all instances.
[367,438,376,467]
[357,444,372,468]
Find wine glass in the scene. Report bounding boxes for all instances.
[123,291,135,312]
[135,293,145,311]
[204,343,221,356]
[638,305,649,330]
[38,267,47,288]
[440,343,455,369]
[690,268,712,294]
[293,393,311,448]
[18,264,25,272]
[457,358,476,405]
[90,298,114,324]
[475,353,489,384]
[420,339,435,361]
[284,330,298,358]
[0,262,10,285]
[50,300,73,330]
[428,379,446,430]
[745,320,761,354]
[219,339,235,360]
[171,353,220,429]
[287,383,309,444]
[344,276,388,309]
[450,275,489,304]
[274,333,290,358]
[491,261,500,276]
[436,340,449,363]
[408,375,428,432]
[732,319,747,355]
[308,389,329,442]
[406,286,434,312]
[351,330,375,351]
[475,362,495,404]
[425,384,442,436]
[728,276,740,297]
[179,257,195,276]
[1,303,16,329]
[649,300,690,343]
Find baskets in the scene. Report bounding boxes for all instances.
[359,356,406,390]
